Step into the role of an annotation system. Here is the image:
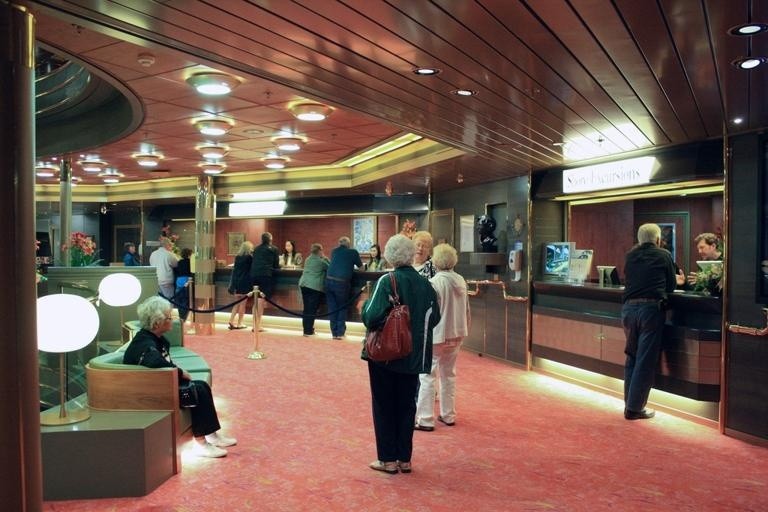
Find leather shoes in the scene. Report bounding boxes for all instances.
[624,408,656,419]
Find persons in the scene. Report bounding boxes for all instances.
[124,242,140,267]
[122,295,236,459]
[228,241,252,329]
[250,232,279,331]
[673,233,723,295]
[148,237,180,302]
[621,224,677,420]
[298,244,331,335]
[175,248,192,322]
[278,240,302,270]
[365,230,471,472]
[325,237,362,339]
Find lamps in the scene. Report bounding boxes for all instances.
[194,119,233,136]
[82,162,105,172]
[36,295,99,425]
[264,158,287,168]
[98,273,142,345]
[135,156,158,166]
[290,104,333,121]
[35,167,56,177]
[58,178,78,185]
[186,73,240,96]
[200,147,224,158]
[272,139,304,151]
[100,175,119,183]
[201,165,224,173]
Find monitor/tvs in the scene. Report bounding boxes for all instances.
[595,264,619,285]
[542,242,574,276]
[696,258,722,275]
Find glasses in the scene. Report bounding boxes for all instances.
[414,243,431,250]
[165,317,173,321]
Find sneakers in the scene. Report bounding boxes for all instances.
[333,336,338,340]
[338,335,348,339]
[397,461,412,473]
[304,333,317,336]
[238,325,247,328]
[415,423,433,431]
[369,461,398,474]
[228,326,237,329]
[193,440,227,457]
[205,433,238,447]
[438,414,455,426]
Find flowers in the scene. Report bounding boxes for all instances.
[399,220,418,239]
[695,224,723,296]
[35,240,47,284]
[61,231,104,266]
[160,225,178,253]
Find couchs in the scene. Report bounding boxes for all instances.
[86,317,213,474]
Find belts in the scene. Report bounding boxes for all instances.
[624,297,659,304]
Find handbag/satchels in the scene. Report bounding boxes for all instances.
[179,382,199,408]
[364,304,413,361]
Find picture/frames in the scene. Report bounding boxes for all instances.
[351,215,378,257]
[226,232,245,256]
